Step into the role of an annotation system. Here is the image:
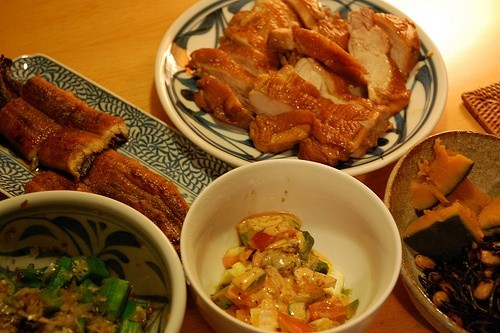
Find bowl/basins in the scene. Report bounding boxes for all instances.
[180,158,402,333]
[382,130,500,333]
[0,190,186,332]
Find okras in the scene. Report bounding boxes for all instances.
[0,256,148,333]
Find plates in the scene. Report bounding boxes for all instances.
[0,53,234,285]
[151,0,448,177]
